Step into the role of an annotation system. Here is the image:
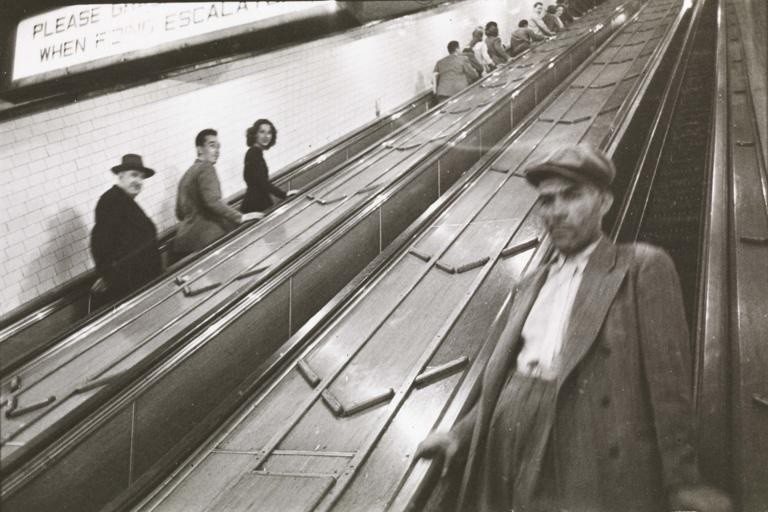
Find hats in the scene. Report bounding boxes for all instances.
[524,143,616,192]
[110,154,155,176]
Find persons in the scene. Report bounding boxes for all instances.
[413,144,734,510]
[432,1,601,105]
[173,129,266,261]
[244,119,298,212]
[90,154,162,305]
